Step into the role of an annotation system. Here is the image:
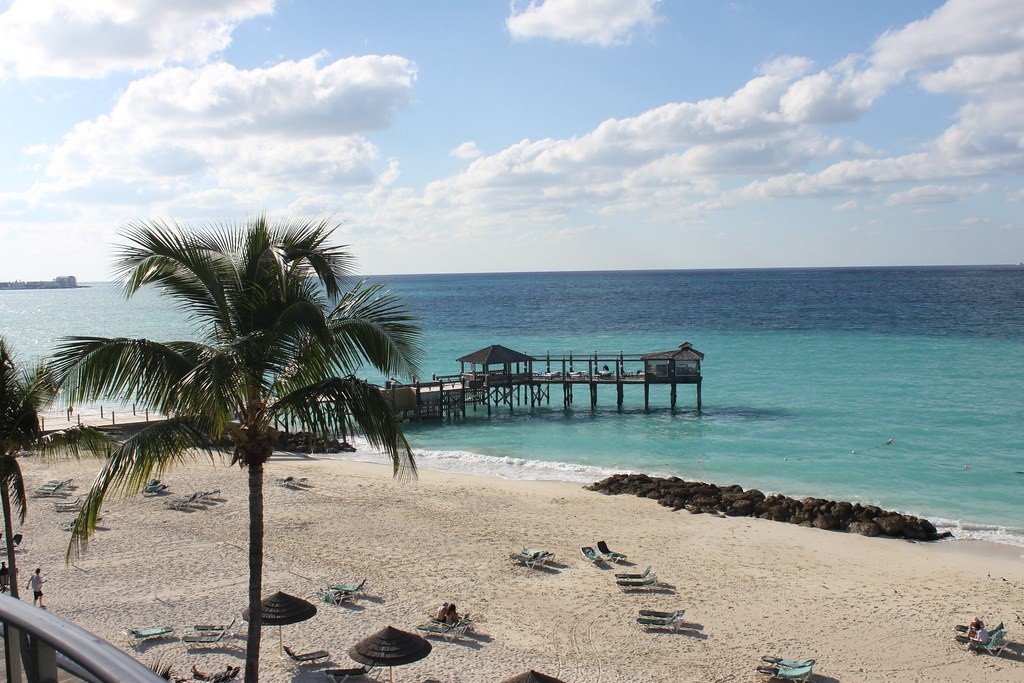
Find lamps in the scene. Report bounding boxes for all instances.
[0,561,10,593]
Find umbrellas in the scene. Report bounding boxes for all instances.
[500,669,566,683]
[242,591,316,656]
[348,626,431,683]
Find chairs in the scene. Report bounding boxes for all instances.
[54,497,103,533]
[277,646,331,677]
[123,625,175,647]
[416,613,474,641]
[0,533,23,555]
[509,547,555,569]
[580,541,627,568]
[637,609,685,632]
[953,620,1009,657]
[325,665,373,683]
[162,489,220,511]
[276,476,308,488]
[143,479,170,497]
[35,478,74,497]
[180,618,243,652]
[186,667,240,683]
[615,566,658,593]
[316,577,366,606]
[755,656,815,683]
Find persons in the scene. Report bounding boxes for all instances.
[27,568,47,608]
[438,602,458,625]
[964,616,990,652]
[603,364,609,372]
[0,562,8,592]
